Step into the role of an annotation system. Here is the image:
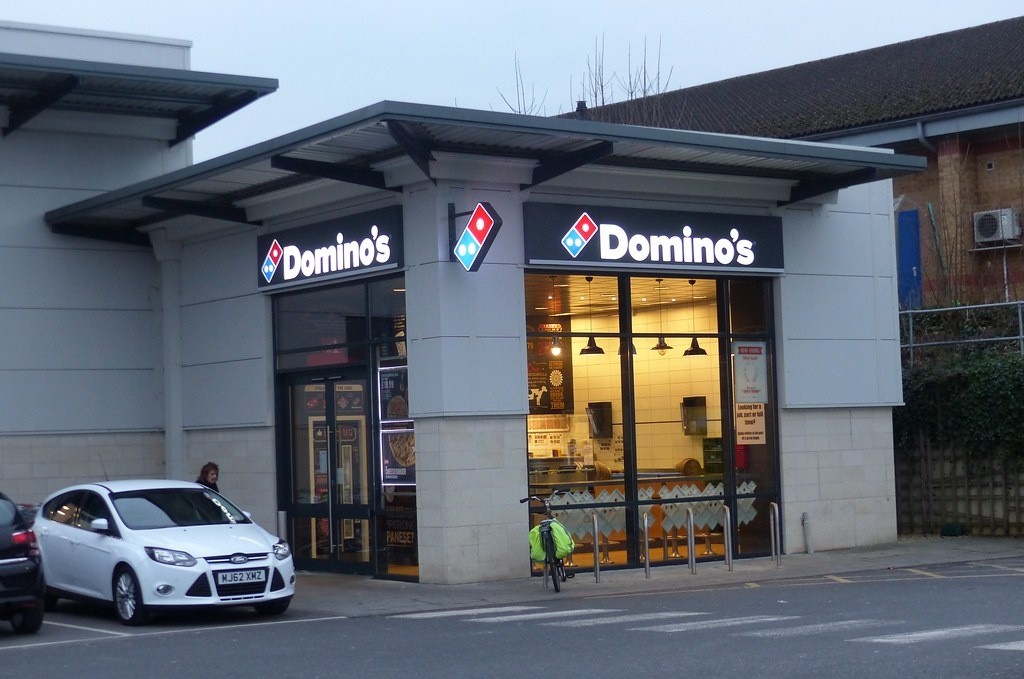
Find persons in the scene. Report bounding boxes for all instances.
[195,462,220,494]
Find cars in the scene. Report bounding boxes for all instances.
[26,477,295,630]
[0,491,46,637]
